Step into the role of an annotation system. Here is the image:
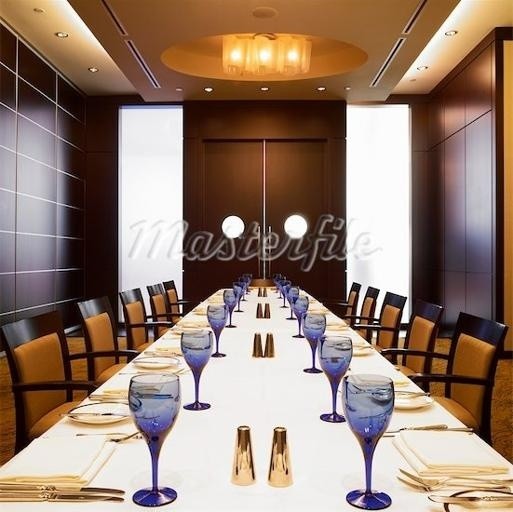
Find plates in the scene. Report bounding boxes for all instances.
[134,356,180,369]
[353,347,375,357]
[66,402,131,425]
[325,326,349,331]
[394,395,433,410]
[175,327,204,335]
[444,489,513,511]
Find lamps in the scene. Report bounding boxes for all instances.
[221,33,312,77]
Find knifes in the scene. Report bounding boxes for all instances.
[395,392,430,397]
[383,428,474,437]
[428,494,513,503]
[0,483,125,494]
[0,492,125,502]
[385,424,448,433]
[60,413,130,417]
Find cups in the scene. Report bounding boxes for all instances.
[233,282,246,312]
[317,336,353,423]
[302,312,327,374]
[223,290,239,328]
[128,374,181,507]
[279,280,291,308]
[275,276,286,299]
[285,286,299,320]
[207,305,228,357]
[243,274,252,292]
[239,277,250,295]
[341,374,395,511]
[273,274,282,293]
[181,330,213,411]
[291,295,309,338]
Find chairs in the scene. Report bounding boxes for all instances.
[354,290,406,364]
[162,278,196,324]
[0,308,101,454]
[76,294,141,394]
[118,287,171,363]
[146,283,172,340]
[380,310,510,424]
[341,286,380,342]
[326,281,362,327]
[388,299,445,394]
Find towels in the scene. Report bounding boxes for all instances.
[0,434,116,489]
[391,429,509,477]
[382,365,411,387]
[103,374,134,394]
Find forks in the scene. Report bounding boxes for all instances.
[398,476,513,492]
[399,468,511,494]
[119,368,185,375]
